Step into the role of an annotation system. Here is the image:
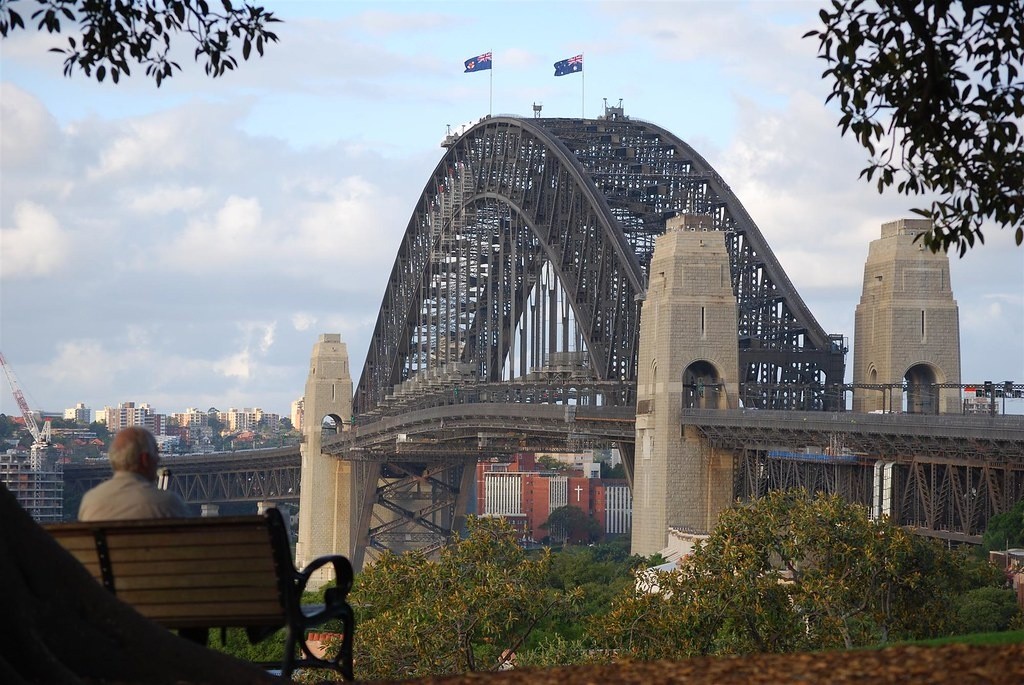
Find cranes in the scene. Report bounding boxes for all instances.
[0,353,53,446]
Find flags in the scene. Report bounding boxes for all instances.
[463,50,492,73]
[553,54,582,76]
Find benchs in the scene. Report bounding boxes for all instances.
[43,508,357,685]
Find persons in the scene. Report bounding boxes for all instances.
[75,426,191,522]
